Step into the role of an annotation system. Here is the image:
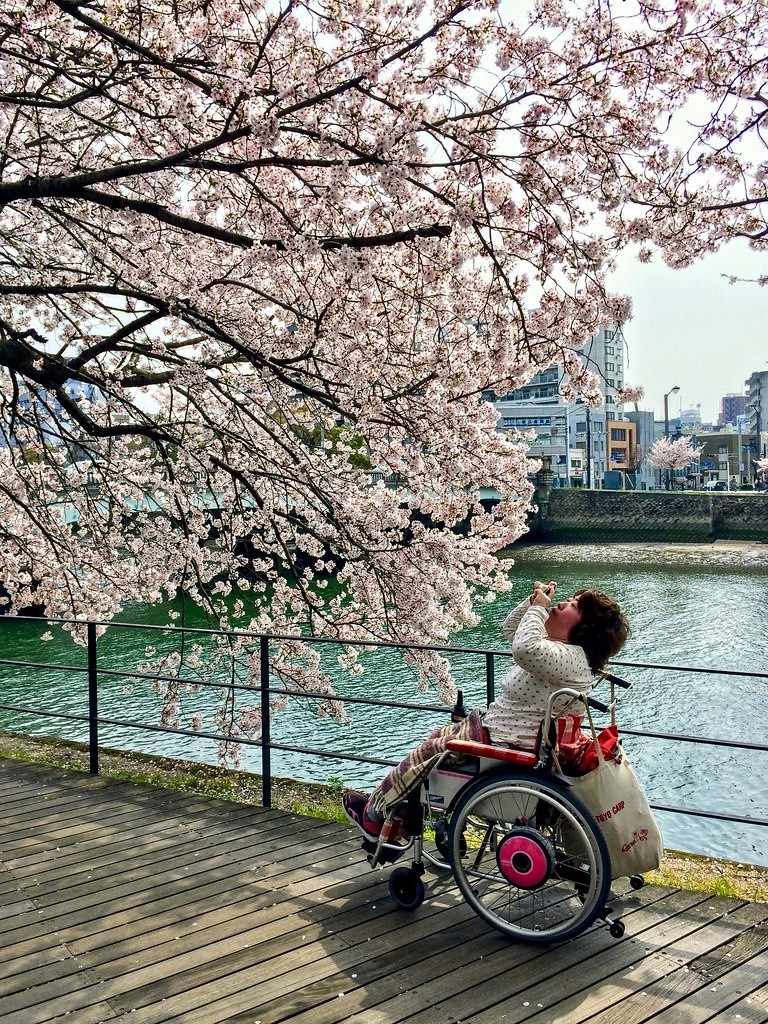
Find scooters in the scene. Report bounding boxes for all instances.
[730,481,737,490]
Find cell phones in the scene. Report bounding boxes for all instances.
[544,585,556,596]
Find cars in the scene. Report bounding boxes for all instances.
[702,481,728,491]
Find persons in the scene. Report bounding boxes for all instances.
[340,579,632,844]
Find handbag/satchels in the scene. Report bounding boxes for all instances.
[542,693,663,882]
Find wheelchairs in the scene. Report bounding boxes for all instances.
[388,667,645,946]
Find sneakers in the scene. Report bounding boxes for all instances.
[342,791,385,843]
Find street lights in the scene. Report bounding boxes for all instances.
[664,385,680,490]
